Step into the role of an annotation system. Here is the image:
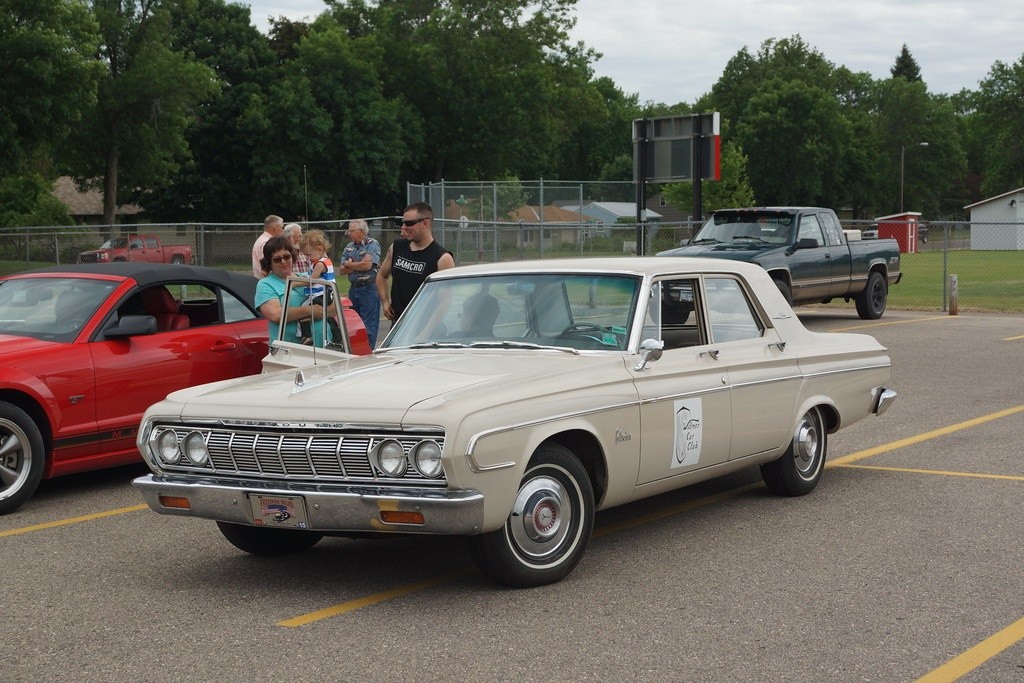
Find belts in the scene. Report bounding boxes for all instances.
[351,282,376,287]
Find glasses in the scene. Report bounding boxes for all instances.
[458,313,463,319]
[401,216,429,226]
[271,253,292,263]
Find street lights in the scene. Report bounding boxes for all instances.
[901,142,929,212]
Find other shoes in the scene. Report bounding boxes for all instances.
[334,337,343,344]
[301,337,313,346]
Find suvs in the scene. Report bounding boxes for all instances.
[918,224,928,244]
[861,223,879,239]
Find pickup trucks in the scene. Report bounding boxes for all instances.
[77,235,193,265]
[654,207,902,320]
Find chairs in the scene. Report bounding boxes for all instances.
[139,286,190,333]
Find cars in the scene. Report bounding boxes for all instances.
[130,256,896,587]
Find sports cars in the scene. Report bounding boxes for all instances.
[0,262,371,516]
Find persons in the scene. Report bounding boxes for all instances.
[375,202,456,334]
[252,215,381,357]
